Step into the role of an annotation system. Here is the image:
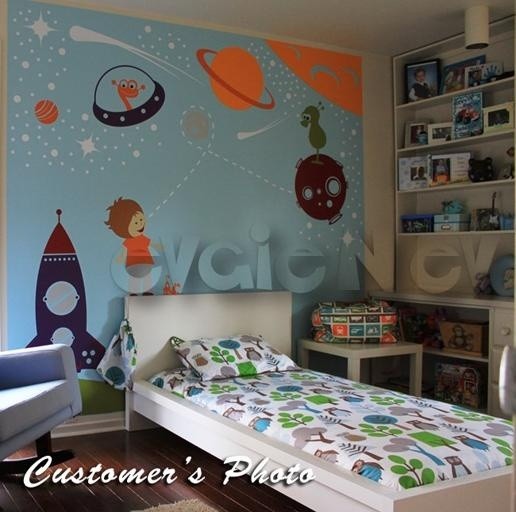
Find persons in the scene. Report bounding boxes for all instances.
[409,68,432,101]
[413,167,427,180]
[434,159,447,181]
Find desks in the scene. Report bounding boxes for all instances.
[295,336,424,397]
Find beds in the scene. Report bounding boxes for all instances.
[124,292,516,512]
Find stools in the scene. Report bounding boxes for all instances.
[0,343,83,475]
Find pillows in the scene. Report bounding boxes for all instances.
[169,335,304,381]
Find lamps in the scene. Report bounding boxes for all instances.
[463,6,490,50]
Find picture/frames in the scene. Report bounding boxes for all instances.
[451,92,482,139]
[404,120,431,148]
[441,54,486,93]
[427,150,472,187]
[398,156,429,191]
[404,58,441,104]
[427,122,451,144]
[464,62,504,89]
[481,101,514,134]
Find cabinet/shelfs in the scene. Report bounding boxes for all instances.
[392,13,516,292]
[491,306,516,420]
[367,294,493,413]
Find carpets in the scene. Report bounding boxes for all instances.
[126,498,219,512]
